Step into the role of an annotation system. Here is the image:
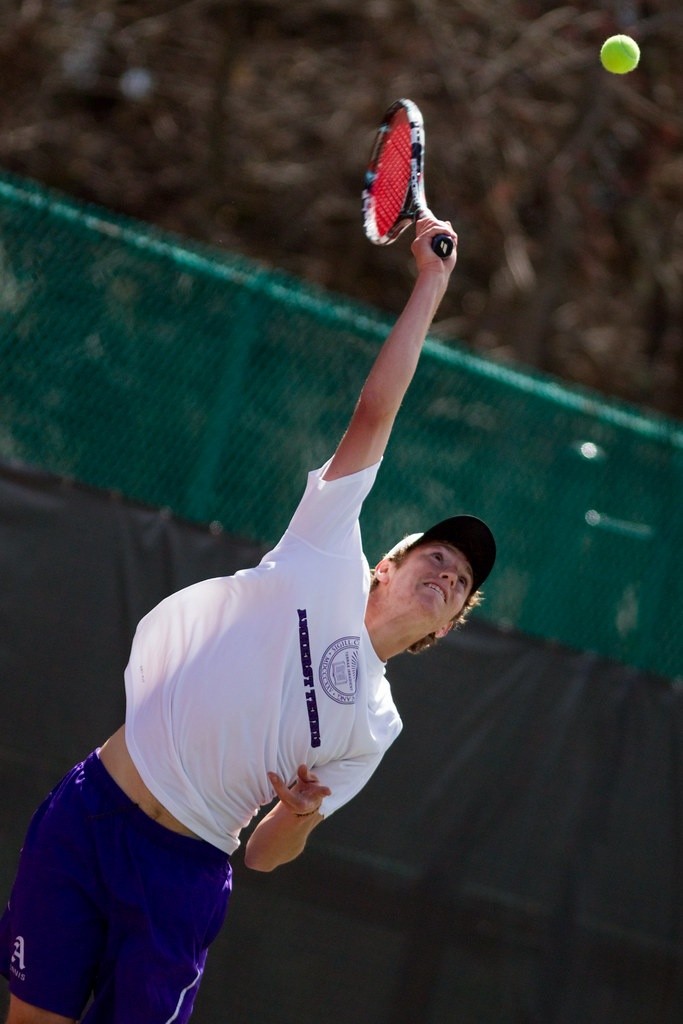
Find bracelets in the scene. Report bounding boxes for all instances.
[296,806,321,816]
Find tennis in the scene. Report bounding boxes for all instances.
[601,34,643,74]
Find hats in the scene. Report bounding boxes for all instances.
[372,514,497,597]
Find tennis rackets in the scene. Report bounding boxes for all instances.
[362,98,453,258]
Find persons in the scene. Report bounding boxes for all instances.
[0,210,497,1024]
